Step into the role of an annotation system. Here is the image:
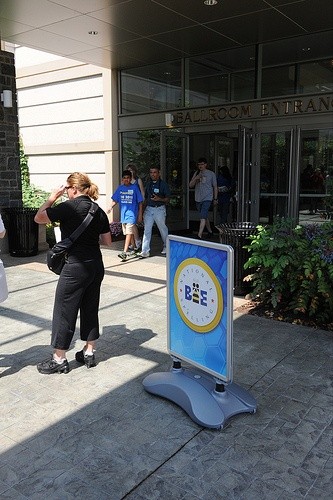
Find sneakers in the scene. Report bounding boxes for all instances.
[75,349,97,369]
[36,358,69,375]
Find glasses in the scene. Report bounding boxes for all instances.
[63,185,73,189]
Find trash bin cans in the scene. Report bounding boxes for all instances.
[0,207,40,258]
[215,220,268,297]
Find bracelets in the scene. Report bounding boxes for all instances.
[213,199,218,200]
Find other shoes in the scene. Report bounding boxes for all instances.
[194,234,200,238]
[160,247,166,256]
[135,246,141,251]
[136,252,150,258]
[129,249,135,255]
[129,245,133,250]
[118,252,129,260]
[207,231,214,238]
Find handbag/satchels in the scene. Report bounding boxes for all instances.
[46,235,74,276]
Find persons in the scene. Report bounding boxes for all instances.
[34,172,112,374]
[107,170,144,260]
[217,166,236,224]
[135,164,171,258]
[0,214,9,304]
[121,164,144,252]
[190,157,217,239]
